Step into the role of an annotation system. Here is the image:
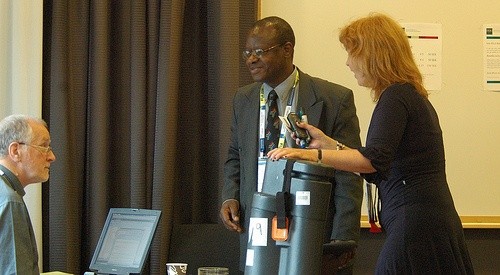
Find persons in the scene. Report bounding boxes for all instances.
[267,16,476,275]
[0,114,55,275]
[219,16,363,270]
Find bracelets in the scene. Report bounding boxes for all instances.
[318,148,322,163]
[337,141,345,150]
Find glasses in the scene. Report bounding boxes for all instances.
[18,142,52,154]
[242,43,287,59]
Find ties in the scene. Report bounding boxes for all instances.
[263,89,283,158]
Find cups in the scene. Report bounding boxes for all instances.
[198,267,229,275]
[166,263,188,275]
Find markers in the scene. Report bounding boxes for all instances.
[279,105,311,148]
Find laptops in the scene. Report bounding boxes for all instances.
[84,208,162,275]
